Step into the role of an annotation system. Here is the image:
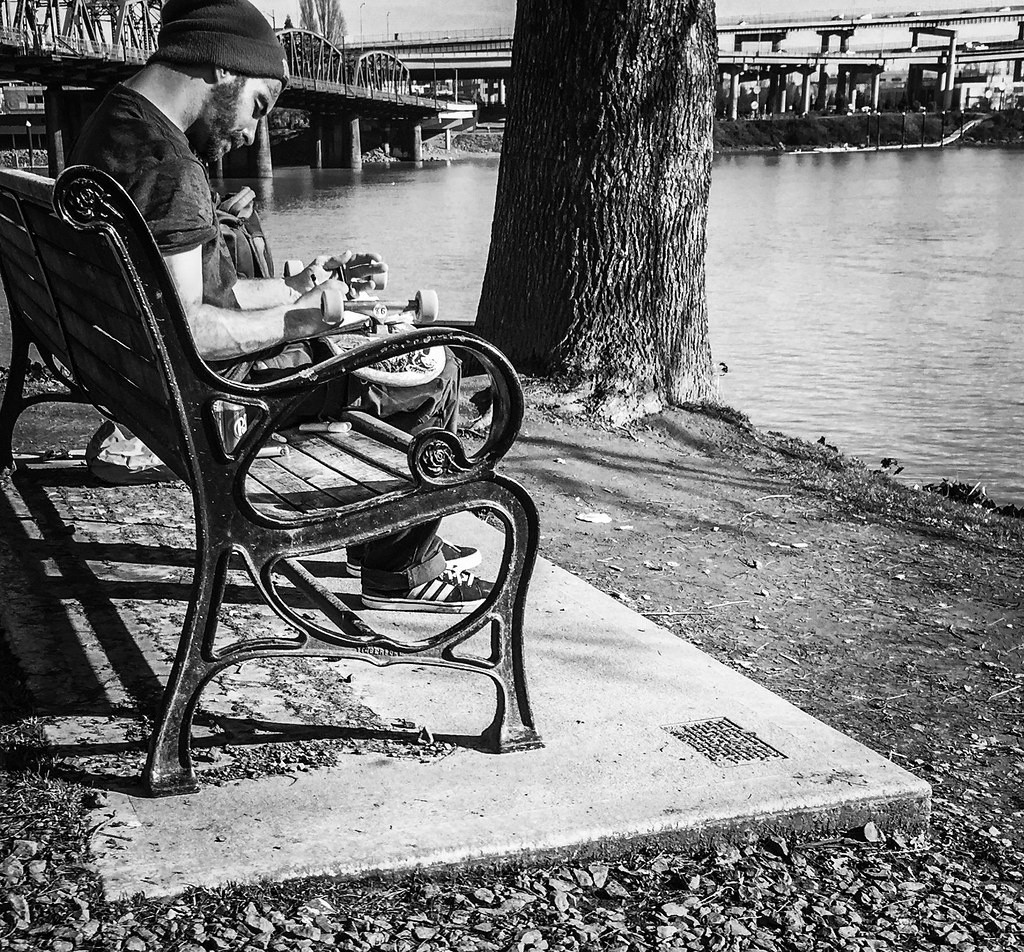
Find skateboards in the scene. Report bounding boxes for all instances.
[283,258,447,388]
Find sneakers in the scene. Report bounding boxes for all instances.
[347,540,482,578]
[361,563,496,614]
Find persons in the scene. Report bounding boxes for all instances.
[71,0,497,615]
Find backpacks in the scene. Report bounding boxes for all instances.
[210,185,274,280]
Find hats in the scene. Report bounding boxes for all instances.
[147,0,289,93]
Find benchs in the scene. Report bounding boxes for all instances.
[0,163,540,798]
